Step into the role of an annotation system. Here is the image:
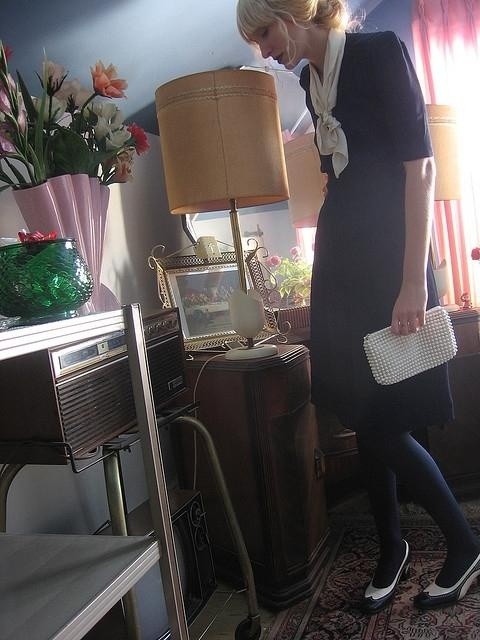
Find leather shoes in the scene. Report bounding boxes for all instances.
[416,546,480,611]
[357,539,410,612]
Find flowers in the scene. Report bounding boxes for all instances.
[0,38,150,192]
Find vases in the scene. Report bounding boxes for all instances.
[0,237,96,319]
[11,172,111,314]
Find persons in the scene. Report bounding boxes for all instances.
[237,0,479,614]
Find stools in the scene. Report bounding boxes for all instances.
[1,399,263,638]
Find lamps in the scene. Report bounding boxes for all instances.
[155,68,291,363]
[283,132,332,227]
[415,102,462,313]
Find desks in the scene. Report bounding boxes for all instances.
[0,304,191,635]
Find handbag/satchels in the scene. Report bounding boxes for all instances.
[362,306,463,385]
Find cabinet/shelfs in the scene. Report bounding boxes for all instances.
[183,341,333,605]
[0,532,179,639]
[417,307,479,507]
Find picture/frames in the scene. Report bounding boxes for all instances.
[147,237,292,355]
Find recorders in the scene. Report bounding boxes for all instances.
[0,307,190,465]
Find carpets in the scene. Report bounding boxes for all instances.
[260,513,480,638]
